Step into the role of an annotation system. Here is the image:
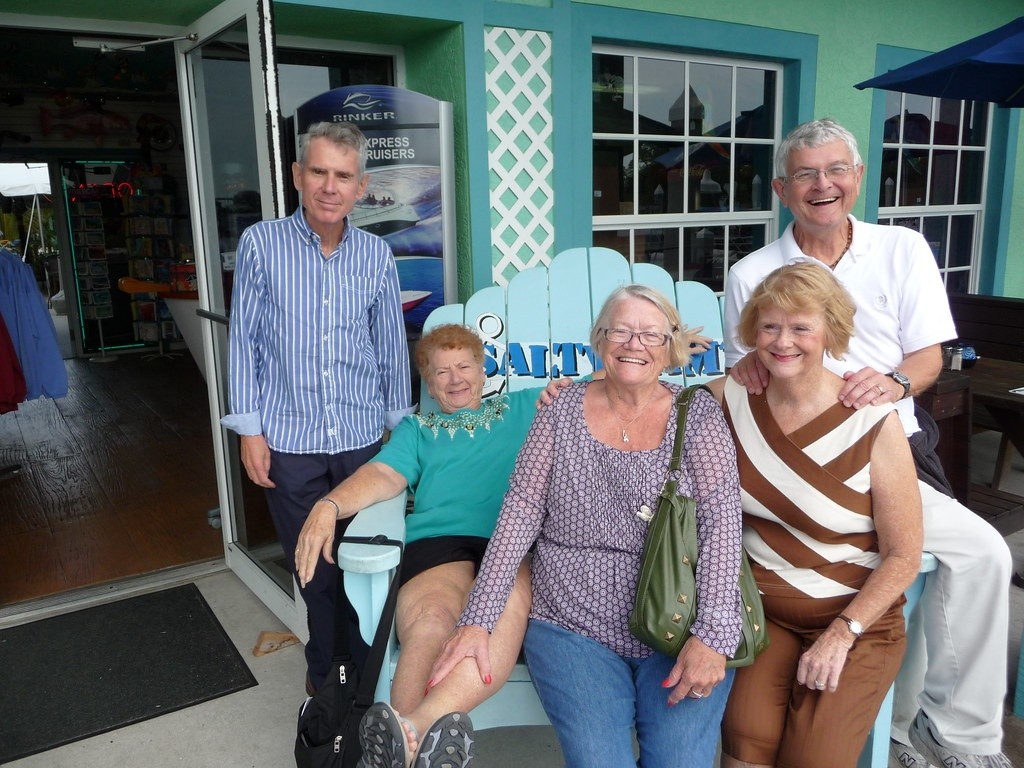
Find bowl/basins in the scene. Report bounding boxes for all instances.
[950,357,977,369]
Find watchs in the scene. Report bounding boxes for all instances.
[884,370,910,400]
[838,614,864,636]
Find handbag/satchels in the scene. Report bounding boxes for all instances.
[295,660,360,768]
[632,384,770,667]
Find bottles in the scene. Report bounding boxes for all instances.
[942,346,963,371]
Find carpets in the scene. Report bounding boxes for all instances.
[0,580,259,765]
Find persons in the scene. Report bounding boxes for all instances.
[533,265,924,768]
[221,125,418,698]
[423,284,743,768]
[296,326,714,768]
[363,193,394,206]
[723,118,1012,768]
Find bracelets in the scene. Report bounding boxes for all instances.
[320,498,339,518]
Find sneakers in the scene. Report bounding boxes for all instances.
[890,709,1015,768]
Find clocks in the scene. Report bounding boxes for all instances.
[148,120,178,152]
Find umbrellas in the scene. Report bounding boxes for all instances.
[851,16,1024,108]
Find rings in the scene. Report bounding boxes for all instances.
[877,386,885,393]
[296,548,300,552]
[815,681,825,690]
[691,691,704,699]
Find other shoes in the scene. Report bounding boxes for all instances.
[360,701,475,768]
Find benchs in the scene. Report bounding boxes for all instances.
[945,290,1024,492]
[339,245,941,768]
[913,371,1024,537]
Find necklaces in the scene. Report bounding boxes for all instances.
[827,221,853,269]
[604,381,658,443]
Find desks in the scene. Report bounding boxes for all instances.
[943,353,1024,492]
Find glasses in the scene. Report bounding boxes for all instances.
[784,165,855,182]
[600,325,671,347]
